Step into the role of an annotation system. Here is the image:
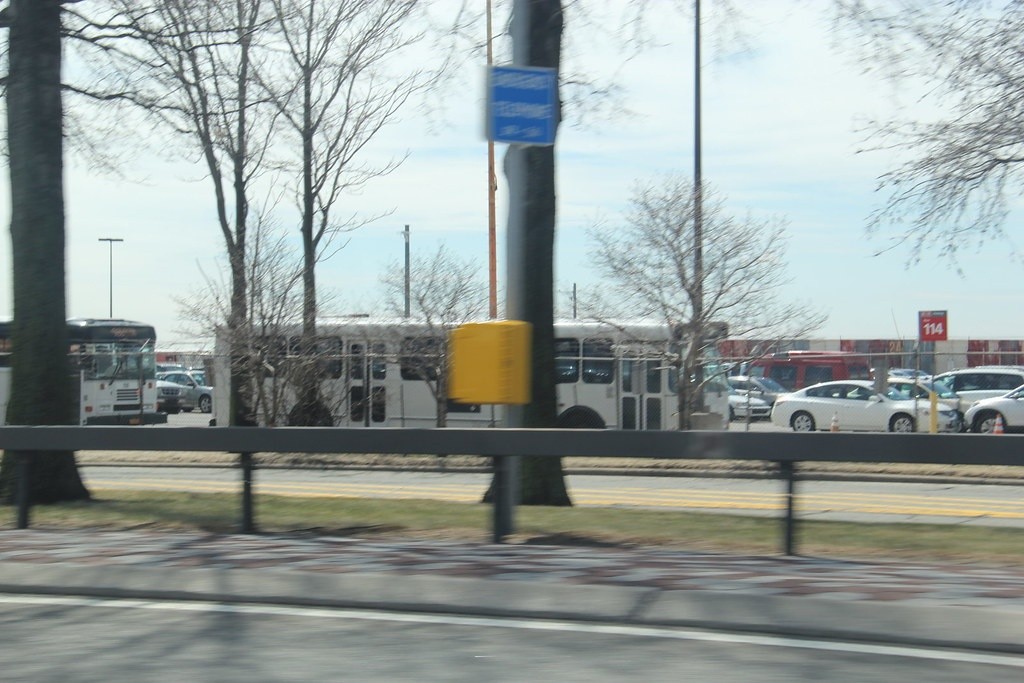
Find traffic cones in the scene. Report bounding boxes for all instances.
[832,411,839,433]
[993,414,1005,433]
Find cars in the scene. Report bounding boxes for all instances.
[728,374,789,423]
[156,363,213,414]
[770,379,962,436]
[831,365,1024,434]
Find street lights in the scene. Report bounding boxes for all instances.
[98,239,124,319]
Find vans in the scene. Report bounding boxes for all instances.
[743,351,872,391]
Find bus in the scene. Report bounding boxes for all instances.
[210,315,734,429]
[1,319,169,427]
